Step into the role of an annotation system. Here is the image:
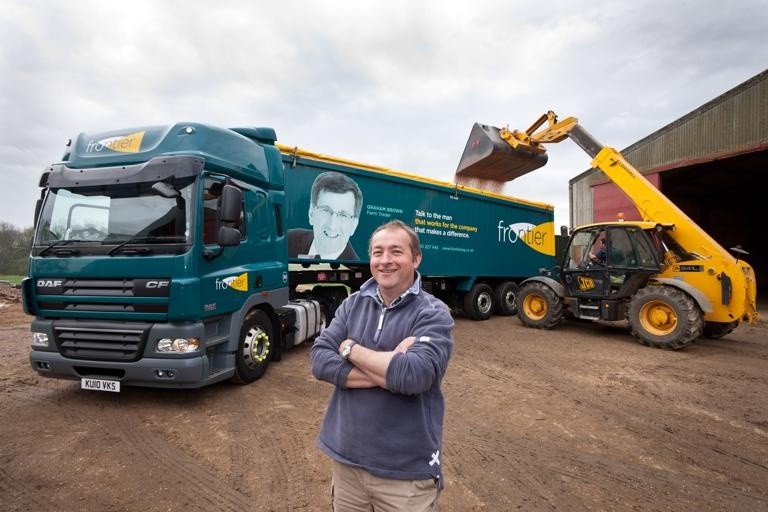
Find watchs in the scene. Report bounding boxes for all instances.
[342,341,358,360]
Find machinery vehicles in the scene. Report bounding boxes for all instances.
[455,111,759,350]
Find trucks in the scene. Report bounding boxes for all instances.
[21,121,560,390]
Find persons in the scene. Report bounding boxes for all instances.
[588,238,613,268]
[310,220,454,512]
[287,171,364,260]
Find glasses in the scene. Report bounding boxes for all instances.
[315,204,356,222]
